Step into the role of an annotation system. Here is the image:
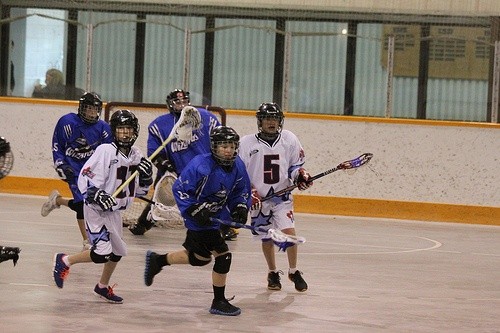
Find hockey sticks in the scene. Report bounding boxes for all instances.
[209,217,306,252]
[259,153,374,203]
[138,196,185,221]
[109,106,201,200]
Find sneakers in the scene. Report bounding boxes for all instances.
[41,190,61,217]
[288,269,307,292]
[92,283,124,304]
[144,250,162,286]
[81,240,92,252]
[52,253,70,288]
[268,270,284,290]
[209,296,241,315]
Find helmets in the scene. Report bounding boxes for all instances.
[78,91,103,124]
[166,89,191,117]
[0,136,13,179]
[209,126,240,169]
[256,102,285,140]
[109,110,140,147]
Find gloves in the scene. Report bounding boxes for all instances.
[293,168,313,191]
[85,186,118,212]
[231,203,249,229]
[250,185,262,212]
[152,154,175,175]
[186,202,216,227]
[136,158,153,187]
[54,158,75,183]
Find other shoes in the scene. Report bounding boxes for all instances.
[128,222,150,235]
[225,228,238,241]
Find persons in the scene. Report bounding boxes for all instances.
[33,69,65,99]
[127,89,224,235]
[10,39,15,96]
[40,91,113,251]
[0,137,22,268]
[53,109,157,305]
[145,125,253,317]
[236,102,312,293]
